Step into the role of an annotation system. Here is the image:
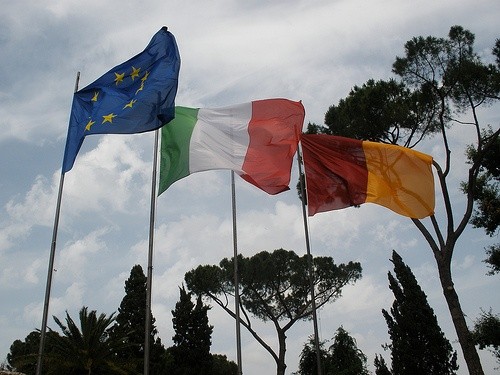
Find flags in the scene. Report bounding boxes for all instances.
[157,99,306,199]
[60,25,182,174]
[299,132,437,219]
